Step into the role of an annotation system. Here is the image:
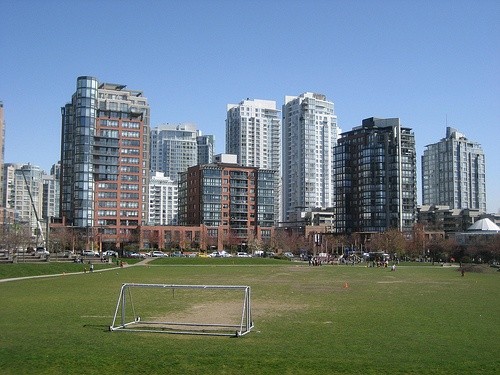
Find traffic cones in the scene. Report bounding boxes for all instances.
[344,282,349,288]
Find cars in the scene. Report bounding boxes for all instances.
[15,248,391,257]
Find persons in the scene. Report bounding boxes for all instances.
[373,260,375,267]
[308,258,321,266]
[377,260,395,271]
[90,263,94,272]
[461,268,464,277]
[84,267,87,273]
[120,260,124,268]
